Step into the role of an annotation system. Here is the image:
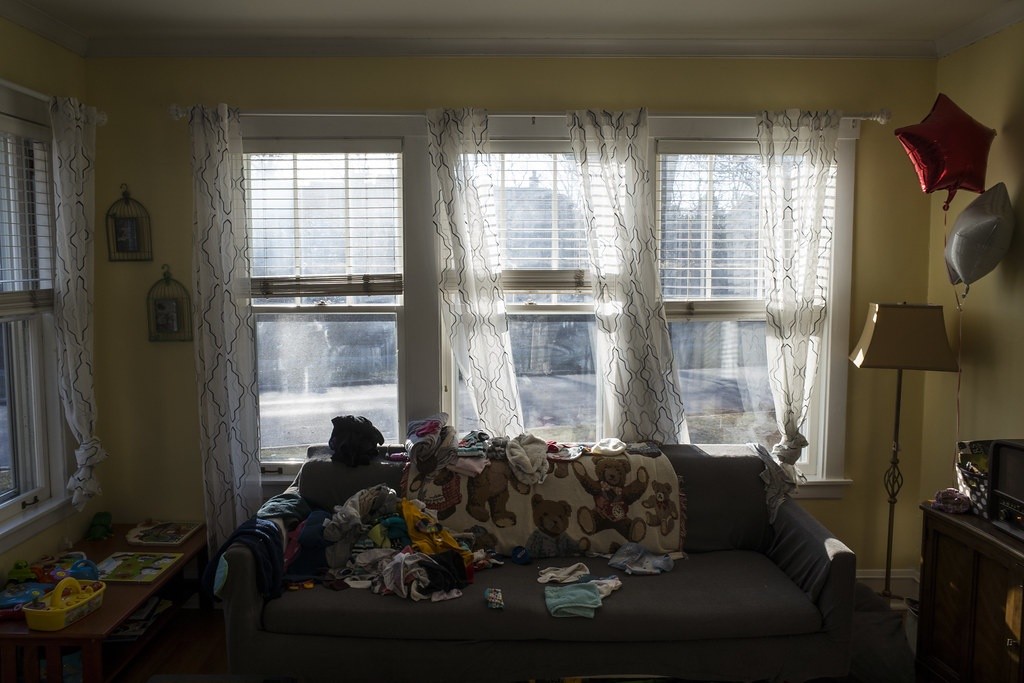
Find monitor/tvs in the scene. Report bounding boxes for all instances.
[986,435,1024,540]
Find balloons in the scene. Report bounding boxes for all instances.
[945,182,1016,286]
[894,94,997,203]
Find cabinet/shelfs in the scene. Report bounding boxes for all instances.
[911,502,1024,683]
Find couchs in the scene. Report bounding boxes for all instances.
[217,446,857,683]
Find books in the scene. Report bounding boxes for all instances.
[96,552,184,583]
[104,597,173,641]
[956,440,992,472]
[126,520,203,546]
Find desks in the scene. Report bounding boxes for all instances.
[0,522,218,683]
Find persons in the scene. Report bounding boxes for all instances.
[156,304,168,326]
[117,220,132,250]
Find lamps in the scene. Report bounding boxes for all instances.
[847,303,962,612]
